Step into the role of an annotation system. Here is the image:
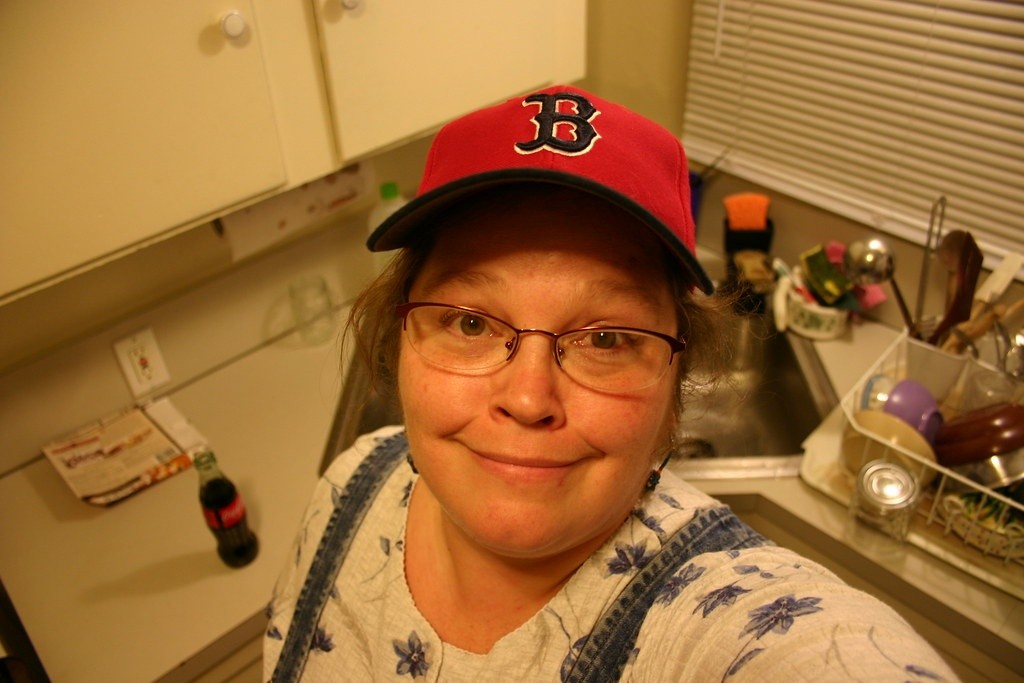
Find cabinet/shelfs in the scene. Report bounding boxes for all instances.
[0,0,587,305]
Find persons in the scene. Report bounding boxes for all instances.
[263,97,966,683]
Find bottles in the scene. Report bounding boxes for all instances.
[193,450,261,570]
[367,182,412,282]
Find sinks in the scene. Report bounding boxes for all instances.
[667,295,837,461]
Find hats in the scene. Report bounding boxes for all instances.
[366,85,714,297]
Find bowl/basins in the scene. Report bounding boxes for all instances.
[861,374,945,446]
[931,484,1024,559]
[841,407,940,489]
[940,403,1024,494]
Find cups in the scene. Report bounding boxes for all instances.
[784,289,849,341]
[962,373,1018,413]
[721,214,777,278]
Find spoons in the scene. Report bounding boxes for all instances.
[1004,345,1024,380]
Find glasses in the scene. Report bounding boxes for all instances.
[392,301,687,393]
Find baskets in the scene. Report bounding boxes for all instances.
[840,327,1024,566]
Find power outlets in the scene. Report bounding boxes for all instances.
[112,324,171,399]
